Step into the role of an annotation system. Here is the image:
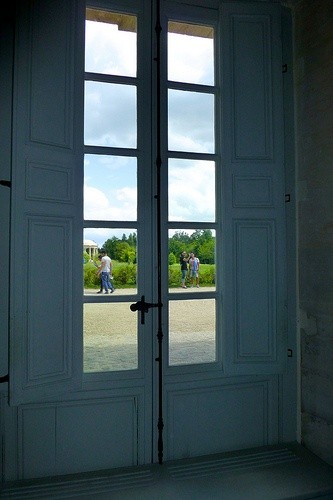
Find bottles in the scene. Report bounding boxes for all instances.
[109,275,113,279]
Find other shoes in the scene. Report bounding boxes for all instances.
[104,291,109,294]
[110,290,114,293]
[97,291,103,293]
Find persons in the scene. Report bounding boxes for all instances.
[180,251,200,289]
[96,251,115,294]
